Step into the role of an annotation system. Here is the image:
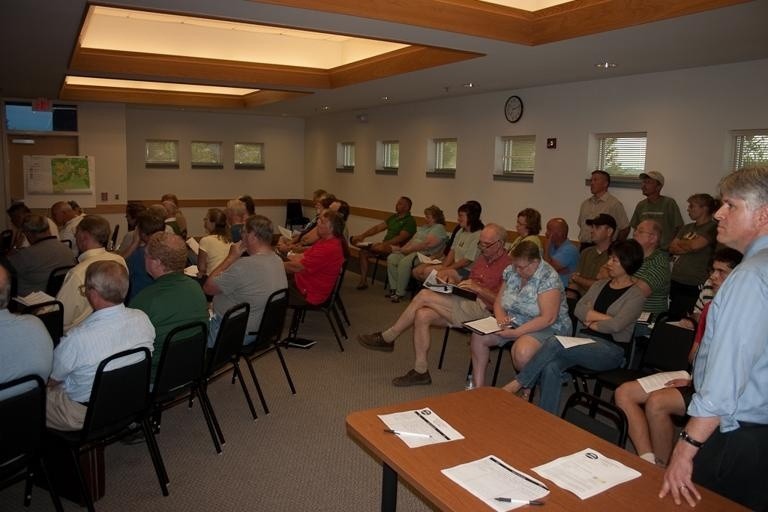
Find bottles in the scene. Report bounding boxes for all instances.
[463,375,476,391]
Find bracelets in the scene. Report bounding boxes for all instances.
[548,258,553,264]
[10,244,20,252]
[587,321,594,328]
[679,429,704,448]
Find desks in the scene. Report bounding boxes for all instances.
[347,385,757,512]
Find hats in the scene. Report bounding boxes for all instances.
[639,171,664,185]
[586,214,616,230]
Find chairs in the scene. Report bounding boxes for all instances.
[560,392,627,450]
[43,265,74,295]
[371,256,388,289]
[565,336,635,413]
[438,325,473,381]
[588,310,697,418]
[492,288,582,404]
[200,303,258,446]
[23,301,65,345]
[145,321,221,486]
[61,240,72,251]
[242,288,297,416]
[0,229,13,256]
[111,225,119,247]
[0,374,63,512]
[291,260,348,353]
[47,347,168,512]
[284,199,310,230]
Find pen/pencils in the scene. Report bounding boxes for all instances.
[499,318,516,326]
[384,430,432,438]
[494,497,543,506]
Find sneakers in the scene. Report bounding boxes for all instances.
[350,236,363,248]
[356,280,368,289]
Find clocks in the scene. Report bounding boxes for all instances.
[505,95,523,124]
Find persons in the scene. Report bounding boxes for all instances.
[188,207,234,303]
[506,208,544,259]
[45,214,130,337]
[114,202,148,231]
[629,170,684,262]
[225,198,251,258]
[161,200,183,238]
[597,219,672,334]
[658,164,767,508]
[161,192,184,216]
[423,203,484,286]
[1,264,55,402]
[541,218,580,292]
[613,247,743,468]
[5,214,73,300]
[501,238,646,417]
[5,200,60,250]
[411,200,482,282]
[128,231,211,394]
[356,223,513,387]
[383,205,450,301]
[47,201,85,261]
[47,260,157,432]
[667,193,722,322]
[241,192,255,219]
[121,207,165,308]
[115,204,176,256]
[202,214,289,349]
[68,200,90,218]
[349,195,417,292]
[567,212,618,336]
[577,169,631,254]
[470,240,573,402]
[278,188,351,307]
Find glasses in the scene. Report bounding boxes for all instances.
[79,284,87,296]
[635,228,653,235]
[477,241,499,251]
[515,263,531,270]
[517,220,527,226]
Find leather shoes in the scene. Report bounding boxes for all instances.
[391,295,405,302]
[393,369,431,386]
[359,332,394,352]
[385,289,396,297]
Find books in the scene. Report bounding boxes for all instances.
[458,316,487,336]
[283,336,318,350]
[635,311,654,325]
[10,289,59,315]
